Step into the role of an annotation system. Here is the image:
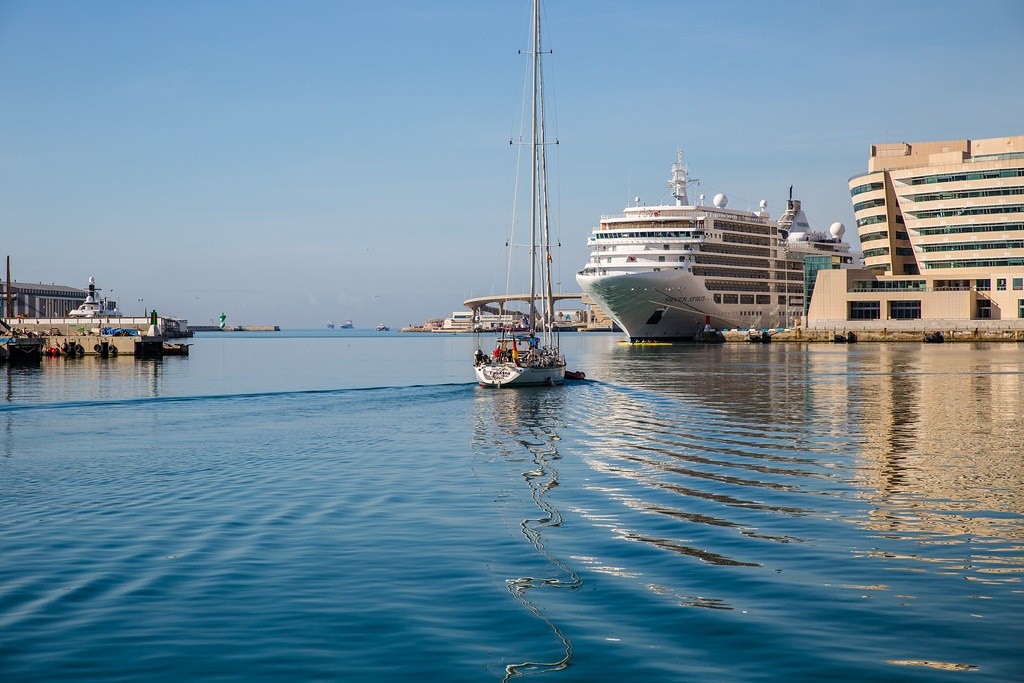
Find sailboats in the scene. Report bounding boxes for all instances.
[474,0,569,389]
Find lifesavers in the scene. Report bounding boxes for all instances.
[94,344,101,352]
[108,344,115,353]
[75,343,83,352]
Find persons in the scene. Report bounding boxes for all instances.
[22,327,27,335]
[10,328,19,338]
[529,331,540,349]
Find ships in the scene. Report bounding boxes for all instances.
[576,144,863,346]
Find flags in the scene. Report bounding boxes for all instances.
[491,344,500,359]
[512,333,522,367]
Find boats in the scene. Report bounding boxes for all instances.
[376,323,390,331]
[327,320,336,329]
[340,319,354,329]
[165,318,194,338]
[0,319,46,362]
